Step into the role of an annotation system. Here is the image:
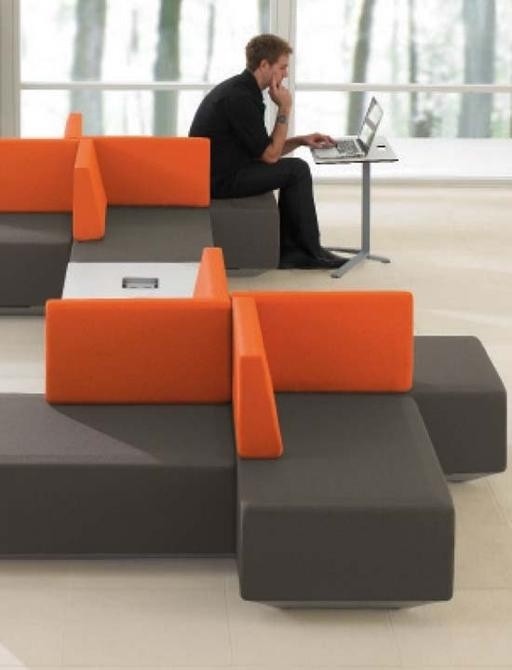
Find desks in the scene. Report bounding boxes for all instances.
[310,135,399,278]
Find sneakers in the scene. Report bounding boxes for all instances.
[279,248,351,270]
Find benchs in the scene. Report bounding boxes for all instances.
[0,247,507,610]
[1,113,281,316]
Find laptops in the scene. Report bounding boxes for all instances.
[312,96,384,159]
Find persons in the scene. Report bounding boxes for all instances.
[187,32,352,271]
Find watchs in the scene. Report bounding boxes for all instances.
[275,113,290,125]
